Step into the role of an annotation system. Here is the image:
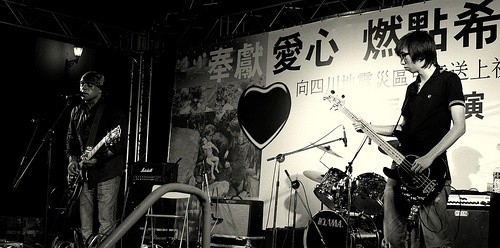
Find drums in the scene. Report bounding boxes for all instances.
[303,209,381,248]
[350,172,388,233]
[313,166,357,212]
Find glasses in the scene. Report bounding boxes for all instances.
[397,51,409,59]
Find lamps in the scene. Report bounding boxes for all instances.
[65,41,84,70]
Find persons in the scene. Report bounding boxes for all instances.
[63,69,122,248]
[352,30,466,248]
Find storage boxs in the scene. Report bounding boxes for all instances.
[7,217,45,246]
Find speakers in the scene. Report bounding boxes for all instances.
[125,161,179,237]
[446,190,500,248]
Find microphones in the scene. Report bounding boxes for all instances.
[62,92,83,100]
[343,127,347,147]
[368,122,371,144]
[30,116,46,123]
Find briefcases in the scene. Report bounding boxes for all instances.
[206,194,262,245]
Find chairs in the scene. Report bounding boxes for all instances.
[140,184,191,247]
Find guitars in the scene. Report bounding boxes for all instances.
[65,125,123,202]
[322,90,449,207]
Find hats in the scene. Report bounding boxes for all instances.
[79,70,105,90]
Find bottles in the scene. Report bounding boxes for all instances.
[493,166,500,193]
